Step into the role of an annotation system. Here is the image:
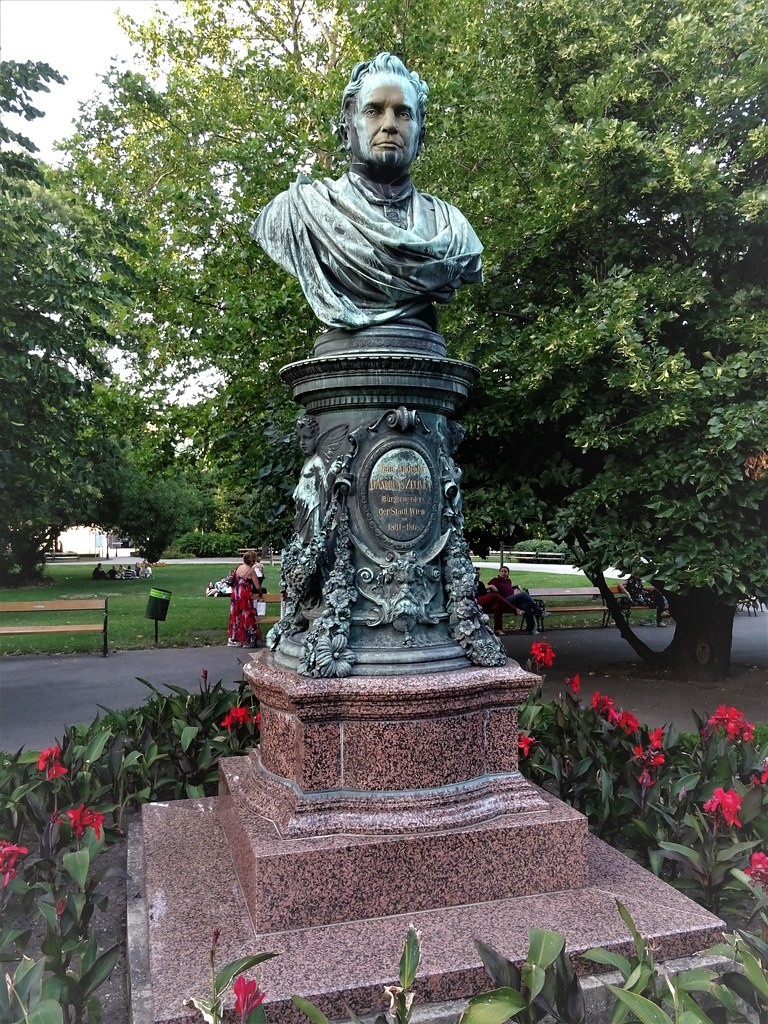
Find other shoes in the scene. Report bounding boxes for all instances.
[660,611,669,617]
[515,608,525,614]
[227,638,242,646]
[541,611,552,617]
[657,621,666,627]
[243,642,256,648]
[495,629,505,635]
[529,628,540,635]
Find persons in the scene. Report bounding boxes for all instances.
[206,570,234,597]
[228,550,263,648]
[251,51,484,332]
[618,575,670,627]
[253,559,265,589]
[474,567,525,636]
[485,566,551,635]
[140,563,152,577]
[92,562,142,579]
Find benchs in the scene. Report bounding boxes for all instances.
[512,587,667,629]
[739,597,757,616]
[0,596,109,657]
[252,594,281,624]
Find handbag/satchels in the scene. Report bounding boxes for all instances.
[224,563,241,588]
[262,573,266,579]
[614,596,632,608]
[256,595,266,615]
